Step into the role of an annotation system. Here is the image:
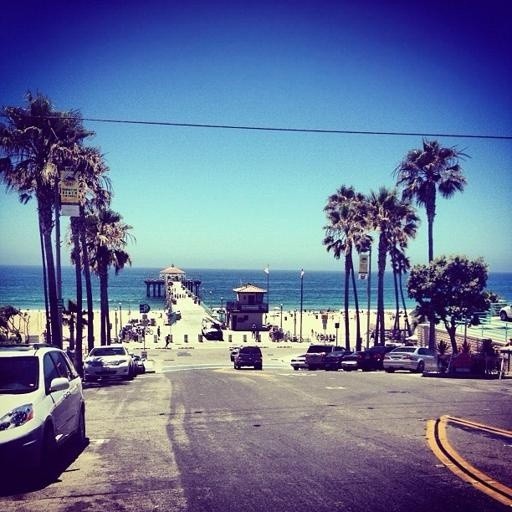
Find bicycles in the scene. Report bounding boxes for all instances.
[145,328,153,335]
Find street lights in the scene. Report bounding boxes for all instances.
[280,303,283,329]
[209,291,211,309]
[118,302,122,331]
[334,317,340,346]
[220,296,223,311]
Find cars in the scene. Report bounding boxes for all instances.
[83,344,145,381]
[201,327,223,340]
[499,303,512,321]
[289,342,439,374]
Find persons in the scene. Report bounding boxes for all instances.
[118,287,402,345]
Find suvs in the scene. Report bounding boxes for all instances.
[0,341,86,472]
[230,345,263,370]
